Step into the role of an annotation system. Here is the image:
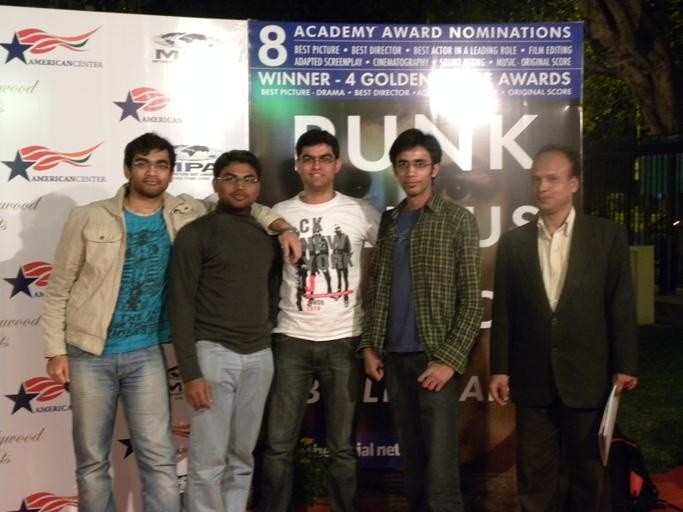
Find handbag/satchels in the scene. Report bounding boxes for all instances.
[610,431,659,512]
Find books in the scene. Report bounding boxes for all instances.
[594,380,622,470]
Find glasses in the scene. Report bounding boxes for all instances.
[301,157,333,164]
[399,161,433,168]
[215,175,259,184]
[133,160,171,170]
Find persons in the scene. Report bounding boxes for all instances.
[327,225,352,306]
[305,225,334,305]
[289,236,311,314]
[167,149,287,512]
[267,126,382,511]
[38,128,302,511]
[308,100,524,512]
[357,127,483,511]
[488,140,640,511]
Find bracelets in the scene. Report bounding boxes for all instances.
[278,225,299,236]
